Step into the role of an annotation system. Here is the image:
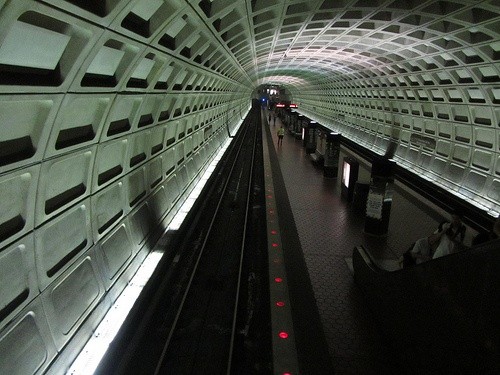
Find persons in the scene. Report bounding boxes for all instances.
[273,112,277,125]
[276,126,285,146]
[268,112,272,124]
[409,234,441,264]
[278,110,305,142]
[430,210,472,260]
